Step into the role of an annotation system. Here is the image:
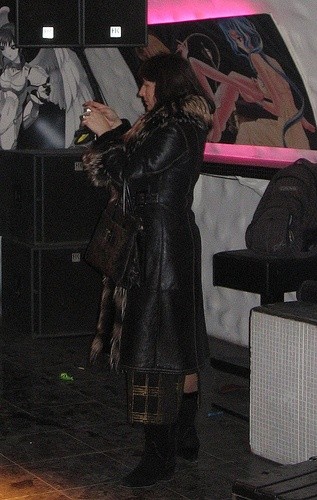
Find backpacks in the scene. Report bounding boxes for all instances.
[245,158,317,259]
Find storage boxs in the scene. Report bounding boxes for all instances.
[0,151,109,244]
[0,237,104,341]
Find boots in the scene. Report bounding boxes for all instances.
[177,390,199,466]
[119,423,171,489]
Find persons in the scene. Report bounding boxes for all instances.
[80,53,211,486]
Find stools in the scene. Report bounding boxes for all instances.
[210,252,317,418]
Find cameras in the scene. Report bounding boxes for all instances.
[82,108,92,118]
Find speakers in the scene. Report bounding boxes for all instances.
[14,0,82,47]
[81,0,148,48]
[0,147,107,247]
[249,301,317,466]
[0,247,104,340]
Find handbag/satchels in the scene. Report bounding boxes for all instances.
[84,179,145,290]
